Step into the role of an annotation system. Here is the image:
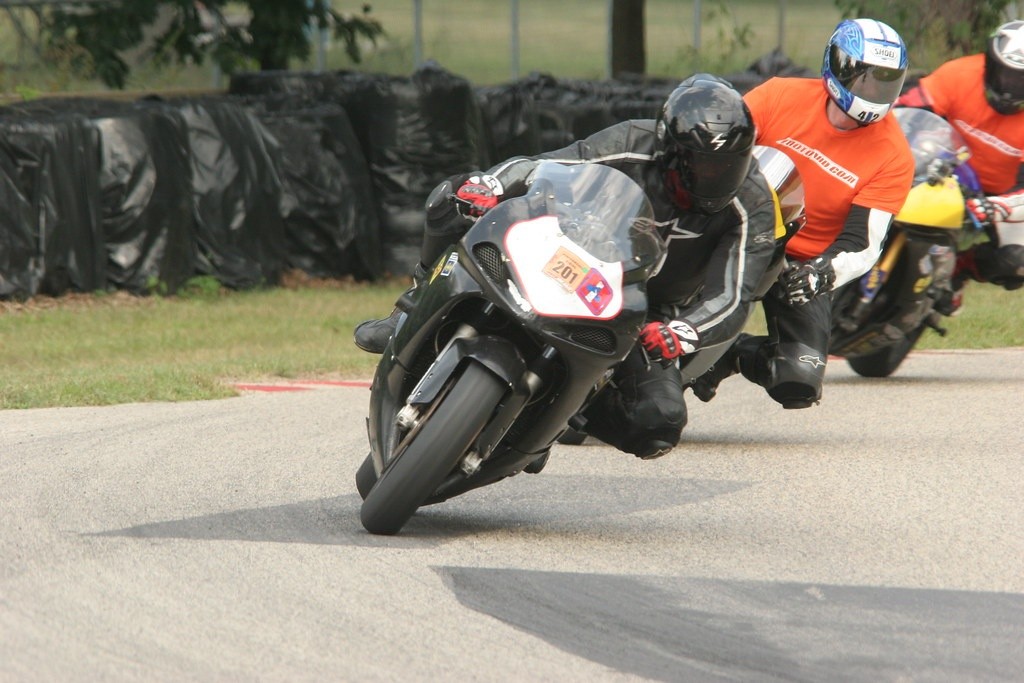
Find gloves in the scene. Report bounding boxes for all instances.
[783,256,836,308]
[639,317,700,361]
[456,174,506,222]
[967,196,1012,228]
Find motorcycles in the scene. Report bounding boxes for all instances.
[354,160,669,535]
[677,142,803,400]
[849,109,1024,377]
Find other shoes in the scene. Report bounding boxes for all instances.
[933,269,964,316]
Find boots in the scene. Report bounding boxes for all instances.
[353,259,430,354]
[686,332,768,403]
[525,444,549,473]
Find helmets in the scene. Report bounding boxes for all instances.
[653,72,755,214]
[821,18,908,123]
[983,20,1024,115]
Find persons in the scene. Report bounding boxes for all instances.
[356,73,776,460]
[688,18,915,410]
[891,20,1024,318]
